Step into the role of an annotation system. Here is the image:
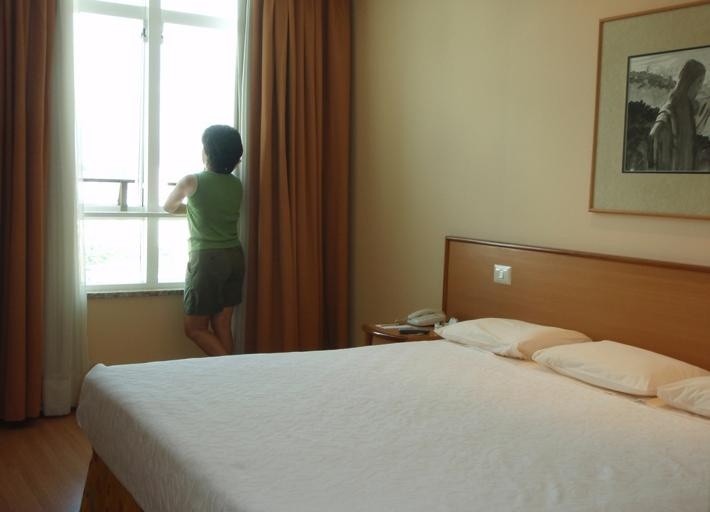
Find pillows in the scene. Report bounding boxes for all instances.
[433,317,592,360]
[531,339,710,397]
[656,375,710,420]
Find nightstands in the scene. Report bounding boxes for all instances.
[361,319,446,345]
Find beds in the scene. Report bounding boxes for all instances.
[75,235,710,512]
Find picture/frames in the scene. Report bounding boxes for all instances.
[588,0,710,220]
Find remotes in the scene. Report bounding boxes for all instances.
[400,328,430,334]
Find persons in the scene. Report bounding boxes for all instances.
[163,124,246,356]
[650,59,706,173]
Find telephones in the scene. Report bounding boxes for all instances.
[407,307,444,327]
